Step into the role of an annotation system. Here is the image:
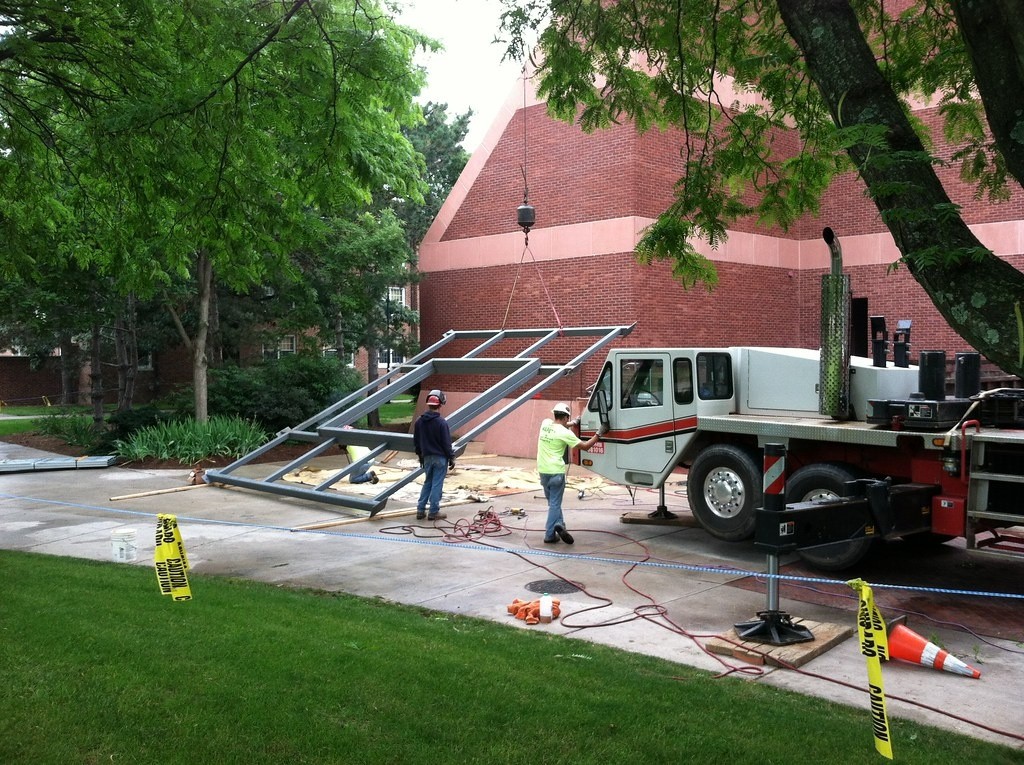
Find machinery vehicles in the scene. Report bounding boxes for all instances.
[566,218,1023,645]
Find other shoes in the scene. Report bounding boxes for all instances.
[544,535,560,543]
[370,471,379,484]
[417,512,426,519]
[428,513,447,521]
[554,525,575,544]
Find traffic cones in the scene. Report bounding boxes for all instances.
[878,616,981,680]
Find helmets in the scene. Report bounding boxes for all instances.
[426,390,446,406]
[550,403,572,416]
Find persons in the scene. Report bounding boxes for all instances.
[414,390,455,520]
[338,425,379,484]
[537,403,610,545]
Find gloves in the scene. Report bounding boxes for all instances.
[449,456,456,470]
[571,416,581,425]
[419,457,425,468]
[596,423,609,436]
